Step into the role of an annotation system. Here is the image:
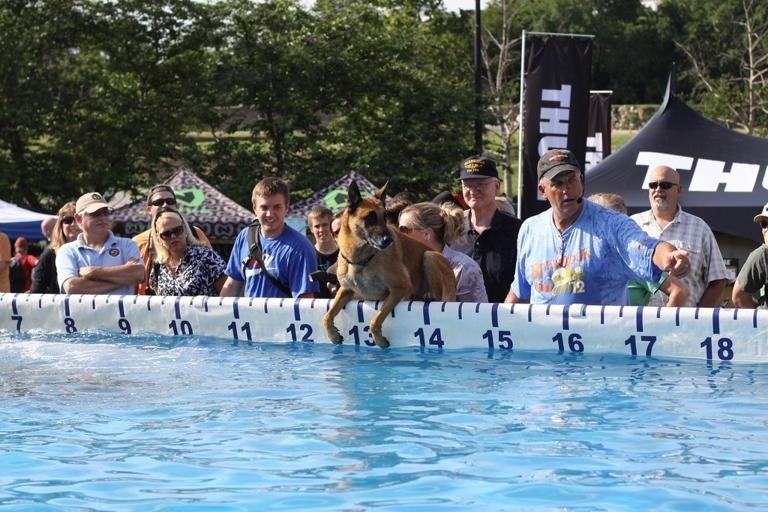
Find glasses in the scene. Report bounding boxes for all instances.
[62,216,74,224]
[150,198,176,206]
[159,226,184,240]
[649,182,677,189]
[400,225,422,233]
[462,181,497,190]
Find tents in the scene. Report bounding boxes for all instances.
[284,170,392,219]
[108,164,257,242]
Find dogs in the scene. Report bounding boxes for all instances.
[322,179,456,349]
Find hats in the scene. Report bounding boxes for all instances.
[754,203,768,222]
[74,191,110,214]
[15,237,29,248]
[457,156,499,181]
[537,149,580,180]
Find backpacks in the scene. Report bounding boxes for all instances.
[9,257,24,293]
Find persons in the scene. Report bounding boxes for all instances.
[388,149,768,308]
[0,177,339,298]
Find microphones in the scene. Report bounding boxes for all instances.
[576,183,585,204]
